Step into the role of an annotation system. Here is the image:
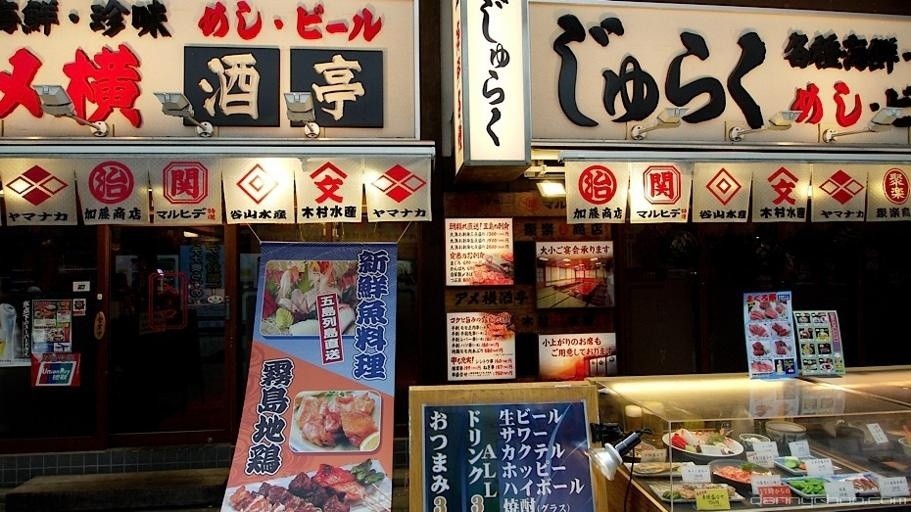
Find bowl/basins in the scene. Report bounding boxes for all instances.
[764,420,807,442]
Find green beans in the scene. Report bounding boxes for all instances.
[790,479,829,497]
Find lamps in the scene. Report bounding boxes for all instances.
[590,427,653,512]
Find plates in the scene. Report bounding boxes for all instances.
[222,390,394,512]
[623,429,884,502]
